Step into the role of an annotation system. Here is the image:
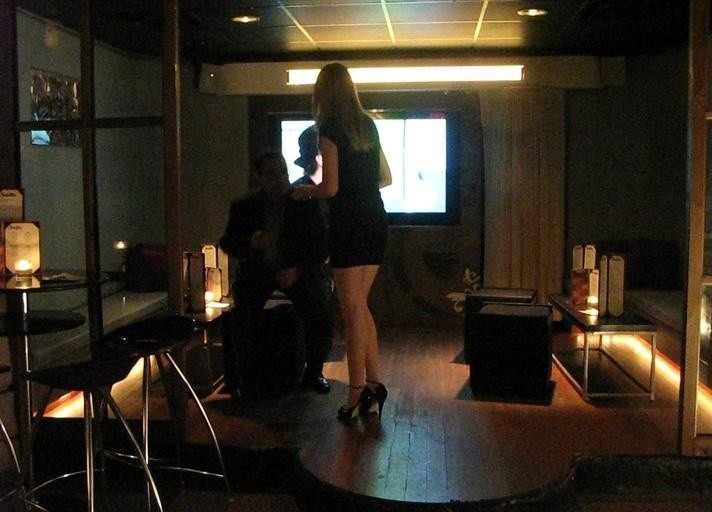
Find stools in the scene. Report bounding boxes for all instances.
[108,314,232,512]
[464,287,536,363]
[474,302,555,402]
[13,354,164,512]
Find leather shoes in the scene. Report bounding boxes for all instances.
[304,374,331,392]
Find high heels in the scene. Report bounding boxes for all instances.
[339,383,387,420]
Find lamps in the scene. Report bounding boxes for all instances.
[14,260,31,278]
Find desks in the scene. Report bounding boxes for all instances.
[0,268,110,511]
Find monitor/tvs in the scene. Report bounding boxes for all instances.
[267,111,462,227]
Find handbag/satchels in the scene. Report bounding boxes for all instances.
[228,305,305,405]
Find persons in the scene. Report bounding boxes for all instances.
[288,63,389,421]
[218,154,331,394]
[290,125,325,187]
